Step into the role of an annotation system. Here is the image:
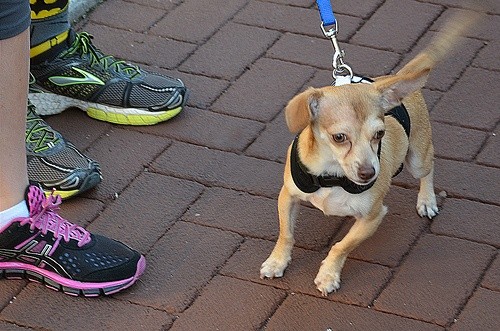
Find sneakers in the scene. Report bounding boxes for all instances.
[1,182,148,299]
[27,31,190,126]
[25,97,105,202]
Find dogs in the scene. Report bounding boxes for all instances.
[257,9,489,298]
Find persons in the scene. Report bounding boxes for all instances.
[0,1,147,296]
[25,1,189,202]
